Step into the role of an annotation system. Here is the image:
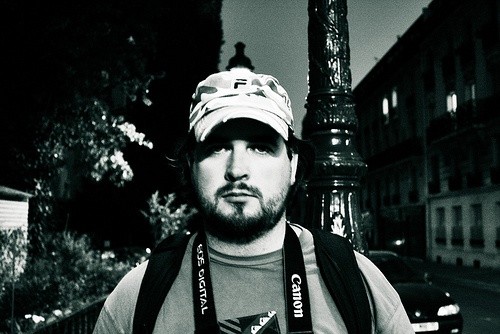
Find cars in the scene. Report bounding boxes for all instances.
[359,250,463,334]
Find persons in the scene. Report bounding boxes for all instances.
[93,66,417,334]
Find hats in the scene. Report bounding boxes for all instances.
[186,69,295,142]
